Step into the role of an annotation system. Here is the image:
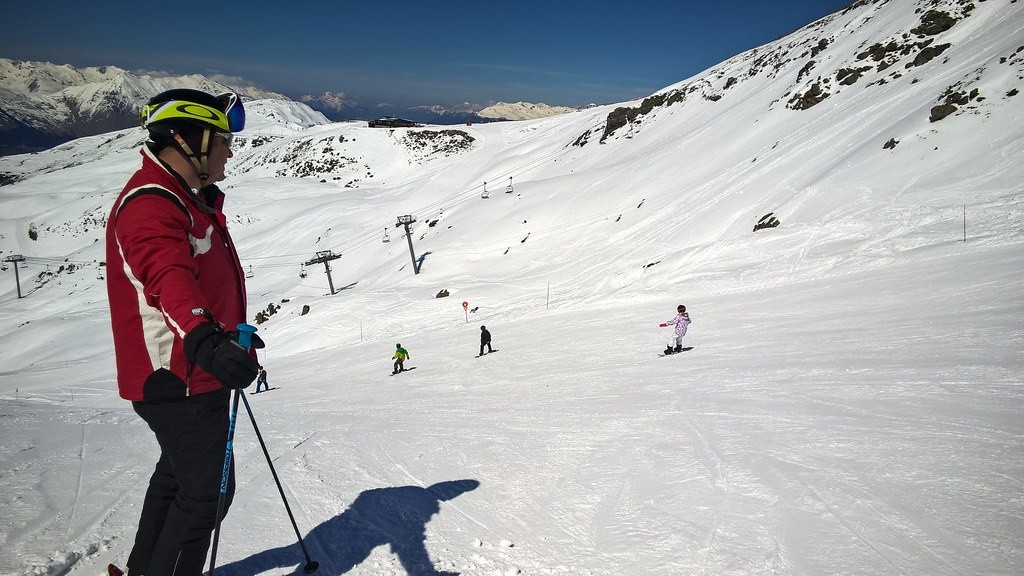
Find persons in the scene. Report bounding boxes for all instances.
[390,343,411,373]
[107,88,262,576]
[255,365,268,393]
[479,325,495,356]
[659,304,692,355]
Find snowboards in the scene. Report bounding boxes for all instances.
[474,349,499,358]
[390,367,416,376]
[658,347,694,356]
[250,387,276,395]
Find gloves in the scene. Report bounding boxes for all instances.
[250,331,265,348]
[659,323,668,328]
[182,320,258,389]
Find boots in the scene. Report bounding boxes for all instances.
[674,344,682,353]
[664,345,673,355]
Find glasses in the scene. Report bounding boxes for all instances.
[214,91,245,133]
[211,131,234,148]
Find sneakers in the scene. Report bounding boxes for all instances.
[393,371,398,374]
[398,368,403,371]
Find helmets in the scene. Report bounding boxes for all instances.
[677,305,686,313]
[141,87,230,132]
